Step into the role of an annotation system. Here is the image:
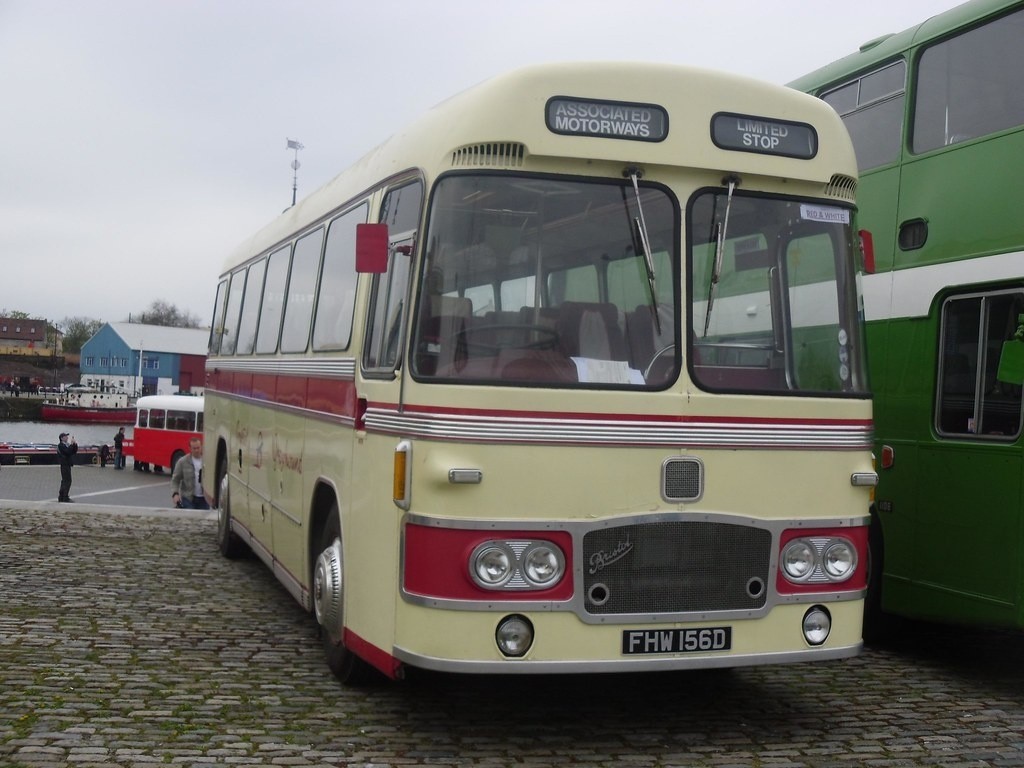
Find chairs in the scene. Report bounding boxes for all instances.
[139,416,203,431]
[415,295,708,385]
[943,370,1022,436]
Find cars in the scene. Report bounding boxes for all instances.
[66,384,91,392]
[39,385,56,392]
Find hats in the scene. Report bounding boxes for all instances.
[59,433,69,439]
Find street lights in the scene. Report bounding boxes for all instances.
[286,140,300,206]
[54,324,58,387]
[138,338,143,398]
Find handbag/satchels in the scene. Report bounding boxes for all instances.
[176,500,184,508]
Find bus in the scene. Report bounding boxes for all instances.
[122,395,204,477]
[201,61,878,687]
[465,0,1024,633]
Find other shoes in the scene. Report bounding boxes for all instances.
[59,495,74,502]
[114,466,124,469]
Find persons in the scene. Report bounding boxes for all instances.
[133,460,165,473]
[57,432,78,503]
[113,427,126,471]
[170,437,210,510]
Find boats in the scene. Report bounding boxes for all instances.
[41,393,172,425]
